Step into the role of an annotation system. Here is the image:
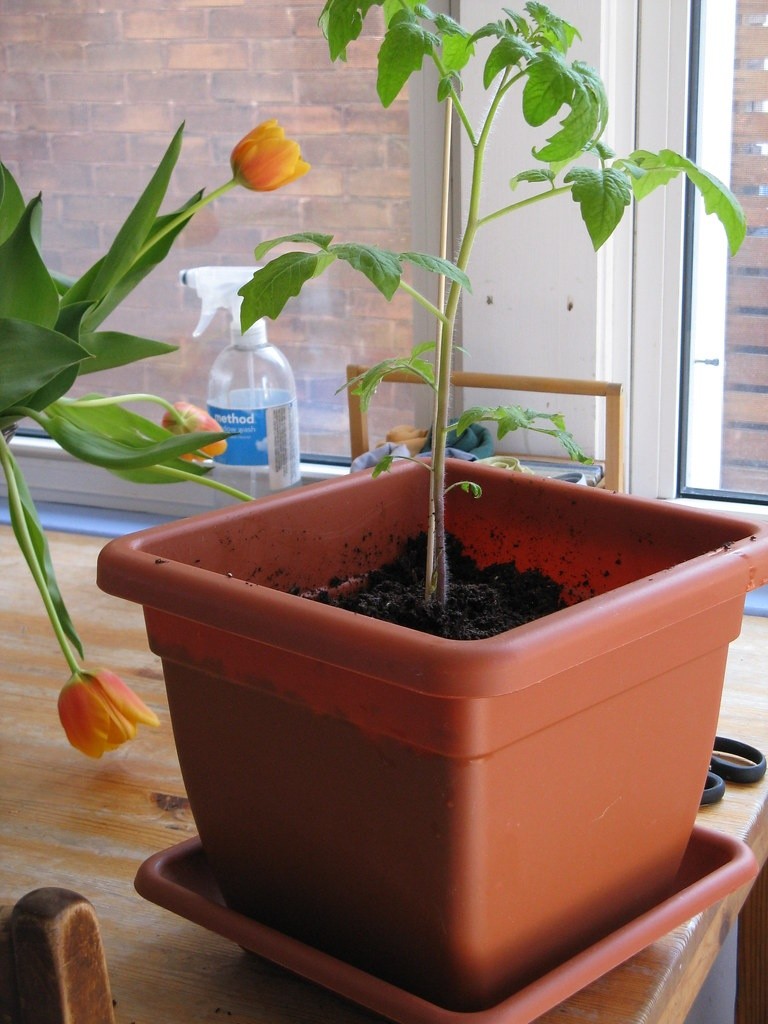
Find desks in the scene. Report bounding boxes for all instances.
[0,523,768,1024]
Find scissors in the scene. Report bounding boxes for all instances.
[700,736,765,804]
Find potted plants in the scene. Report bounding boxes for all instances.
[96,0,768,1024]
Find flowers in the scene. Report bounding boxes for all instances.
[0,120,310,759]
